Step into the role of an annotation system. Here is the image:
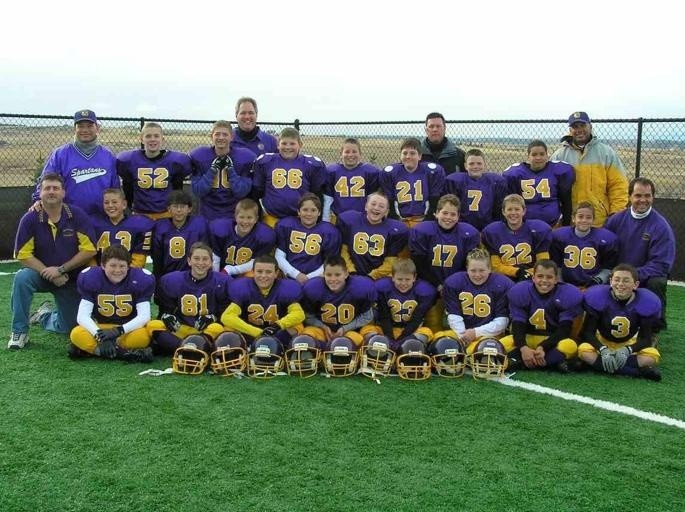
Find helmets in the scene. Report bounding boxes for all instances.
[359,334,401,369]
[428,336,465,372]
[173,333,211,370]
[284,332,321,371]
[248,334,284,373]
[212,330,247,370]
[323,336,360,374]
[470,338,510,375]
[397,338,431,371]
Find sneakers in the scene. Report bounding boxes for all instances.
[6,331,31,351]
[121,346,155,364]
[636,364,664,384]
[27,299,56,325]
[65,342,95,360]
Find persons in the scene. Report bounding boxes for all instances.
[65,242,676,382]
[7,172,675,348]
[29,97,629,228]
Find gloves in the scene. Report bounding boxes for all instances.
[600,348,618,375]
[193,313,216,332]
[98,339,120,361]
[260,325,276,336]
[158,311,180,333]
[515,268,532,283]
[221,154,234,171]
[94,323,125,342]
[583,274,604,290]
[211,155,225,172]
[614,345,631,370]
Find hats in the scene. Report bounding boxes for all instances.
[567,111,592,128]
[73,109,96,125]
